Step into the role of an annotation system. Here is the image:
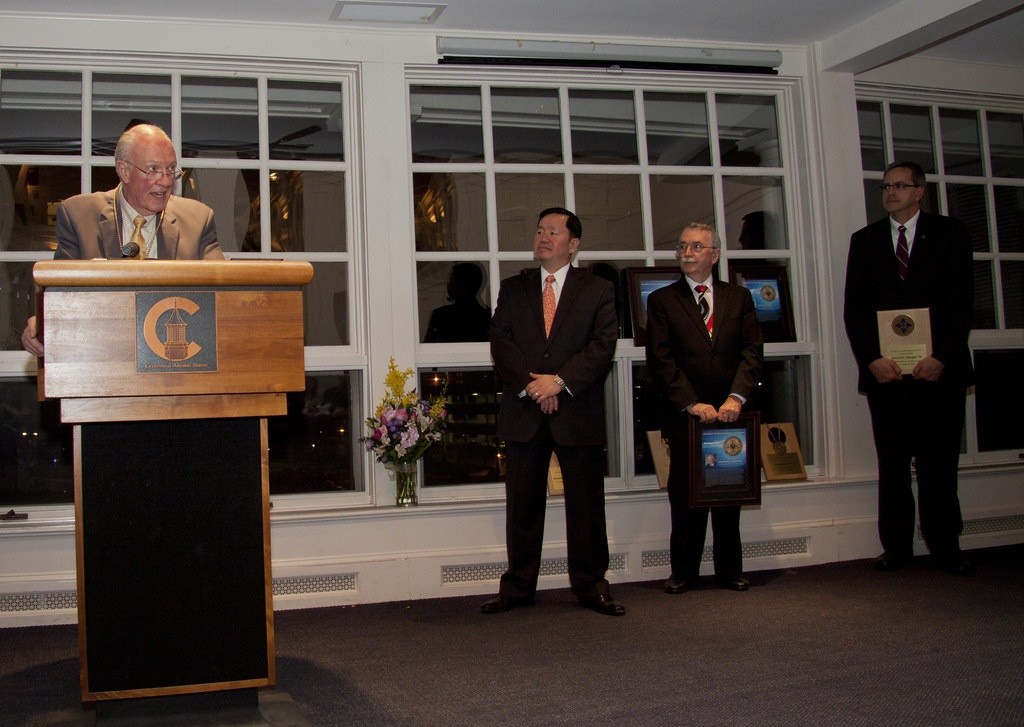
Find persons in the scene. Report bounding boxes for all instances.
[646,223,764,593]
[477,207,625,614]
[22,124,224,357]
[843,163,971,573]
[706,455,716,468]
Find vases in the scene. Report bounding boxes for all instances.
[393,462,418,506]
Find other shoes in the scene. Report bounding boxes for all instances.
[865,553,913,569]
[943,553,969,576]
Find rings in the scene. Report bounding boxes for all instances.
[729,416,733,419]
[535,393,539,397]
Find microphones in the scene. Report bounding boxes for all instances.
[120,242,140,259]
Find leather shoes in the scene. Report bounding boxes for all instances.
[578,593,625,614]
[480,593,533,614]
[664,574,691,593]
[718,579,749,590]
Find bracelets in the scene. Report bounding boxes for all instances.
[554,375,565,390]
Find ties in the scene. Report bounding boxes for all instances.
[130,216,147,261]
[542,275,556,340]
[694,285,714,343]
[894,226,908,281]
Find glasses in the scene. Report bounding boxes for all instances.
[881,182,918,191]
[676,242,716,253]
[123,160,185,183]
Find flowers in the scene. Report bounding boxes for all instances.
[358,355,455,502]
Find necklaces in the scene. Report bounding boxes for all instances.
[114,183,164,258]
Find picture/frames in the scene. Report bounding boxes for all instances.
[727,262,799,343]
[626,265,683,348]
[686,411,760,507]
